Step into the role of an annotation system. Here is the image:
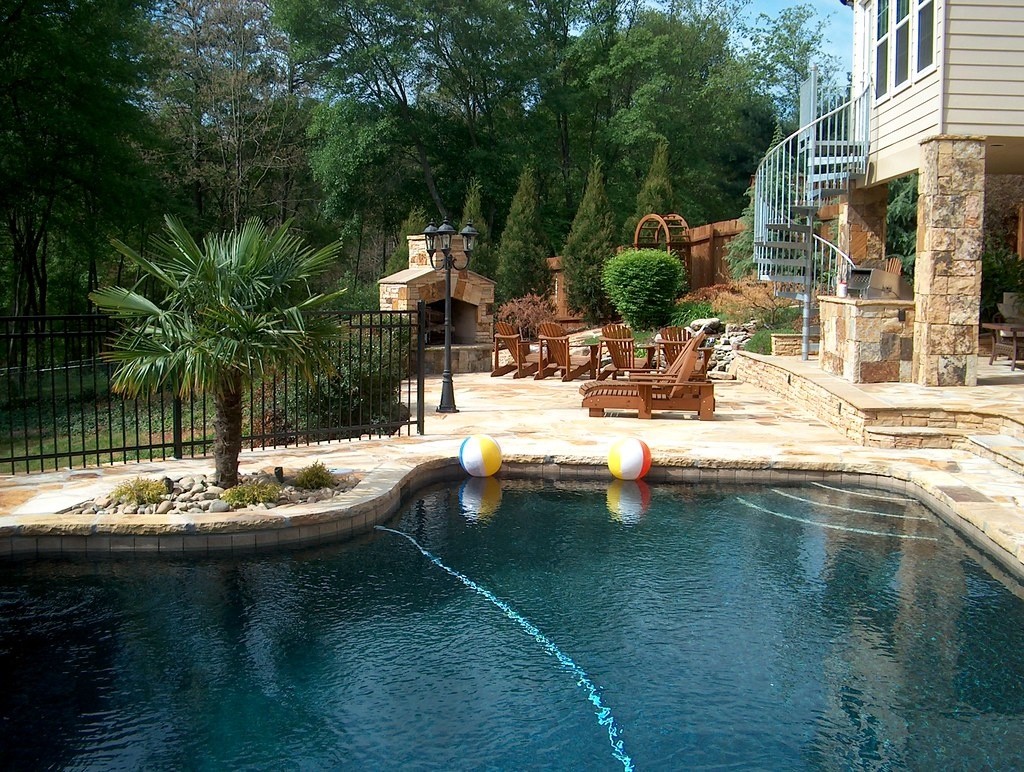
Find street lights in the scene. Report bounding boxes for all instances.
[421,216,479,414]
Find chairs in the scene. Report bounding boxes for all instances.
[492,322,716,421]
[993,292,1024,361]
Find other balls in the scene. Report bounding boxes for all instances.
[607,437,652,480]
[459,435,502,477]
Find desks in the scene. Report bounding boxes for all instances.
[982,322,1024,371]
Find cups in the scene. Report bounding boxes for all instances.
[838,282,848,298]
